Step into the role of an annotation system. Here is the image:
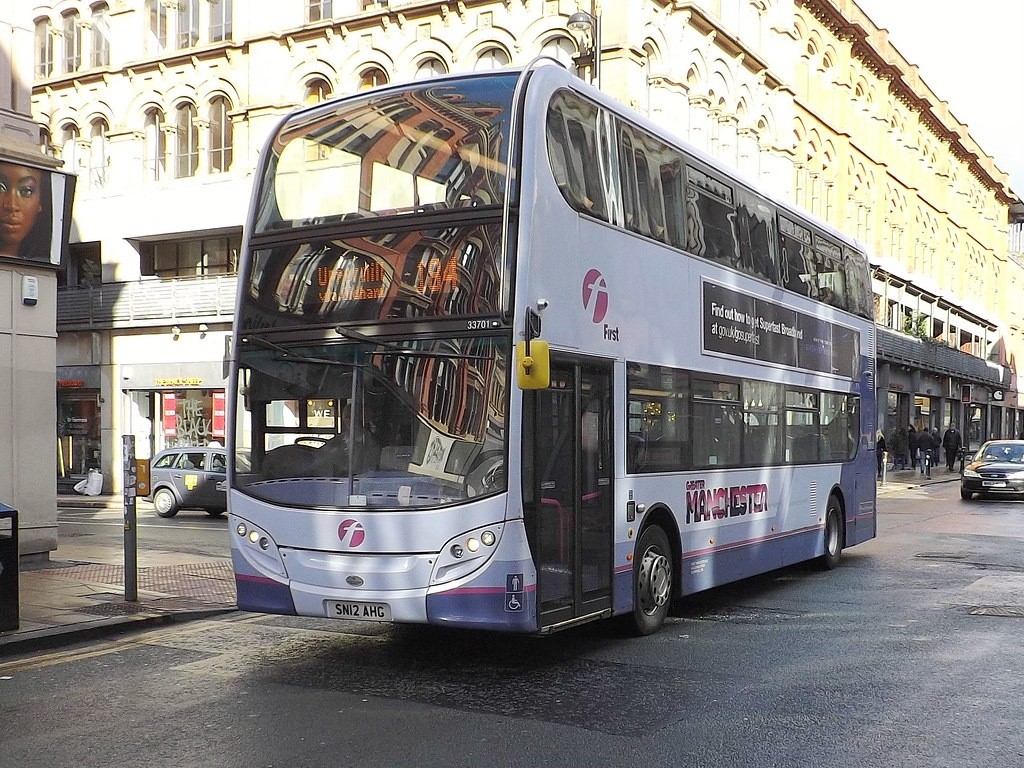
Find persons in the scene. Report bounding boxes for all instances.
[323,402,379,466]
[875,422,963,477]
[0,161,54,257]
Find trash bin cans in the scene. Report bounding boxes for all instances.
[0,502,20,632]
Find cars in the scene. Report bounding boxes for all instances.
[961,439,1024,504]
[145,447,253,519]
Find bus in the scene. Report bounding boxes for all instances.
[225,55,882,639]
